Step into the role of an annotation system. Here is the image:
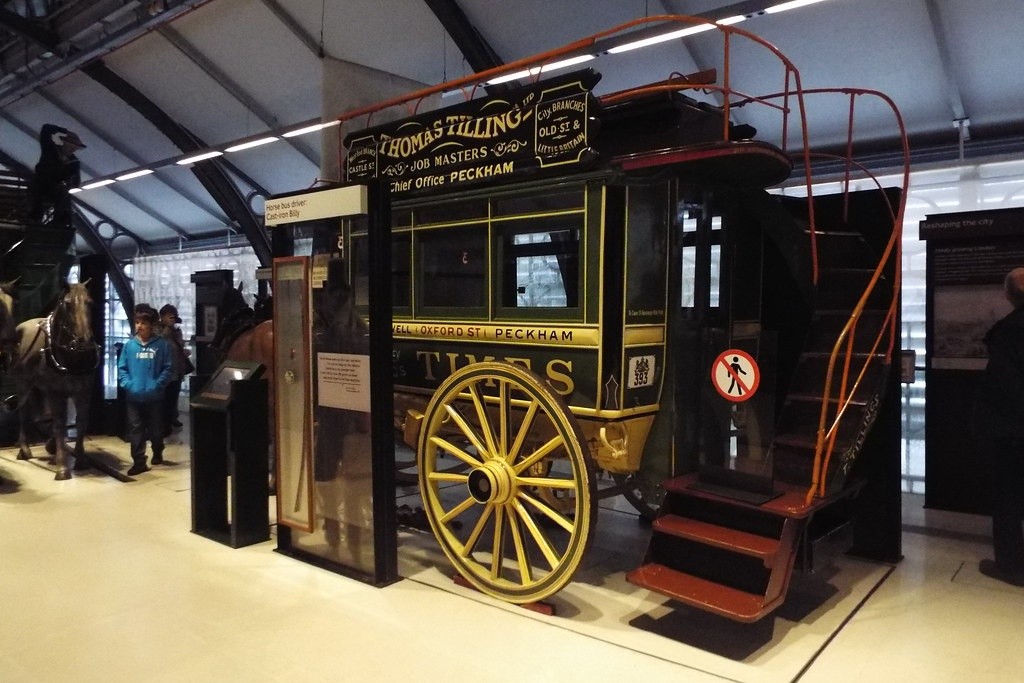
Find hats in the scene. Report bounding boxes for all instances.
[159,304,178,315]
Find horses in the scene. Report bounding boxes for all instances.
[0,274,104,479]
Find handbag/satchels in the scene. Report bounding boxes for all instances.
[184,357,196,375]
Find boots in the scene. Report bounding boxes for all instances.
[127,456,148,476]
[151,445,166,464]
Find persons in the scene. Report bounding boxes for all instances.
[155,305,190,438]
[118,305,177,475]
[27,122,86,227]
[970,267,1024,587]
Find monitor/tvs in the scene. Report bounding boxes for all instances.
[189,359,265,411]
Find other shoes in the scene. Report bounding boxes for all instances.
[171,419,183,427]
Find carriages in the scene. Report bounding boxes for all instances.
[196,15,911,625]
[0,123,96,480]
